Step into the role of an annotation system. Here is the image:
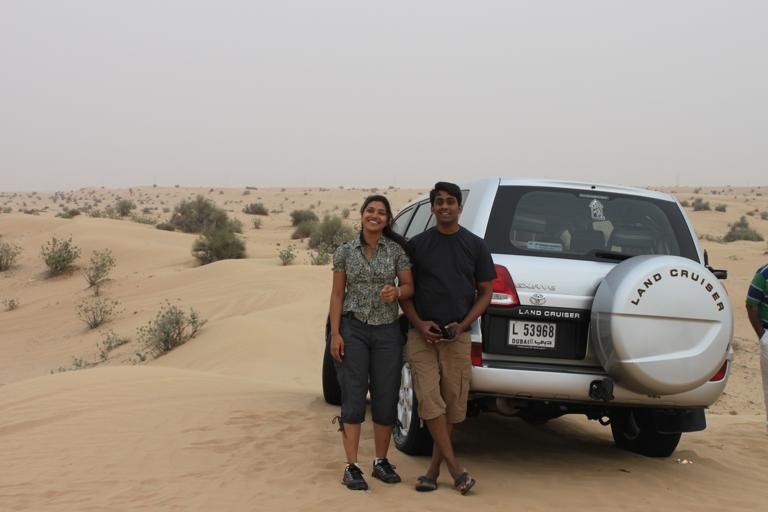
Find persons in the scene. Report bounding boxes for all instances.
[395,181,500,494]
[327,194,417,490]
[745,258,767,426]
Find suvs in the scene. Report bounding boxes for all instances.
[324,176,734,458]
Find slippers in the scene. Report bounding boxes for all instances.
[415,476,437,491]
[454,472,476,495]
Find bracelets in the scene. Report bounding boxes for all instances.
[397,287,401,300]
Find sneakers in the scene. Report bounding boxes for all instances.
[371,460,400,483]
[342,464,368,490]
